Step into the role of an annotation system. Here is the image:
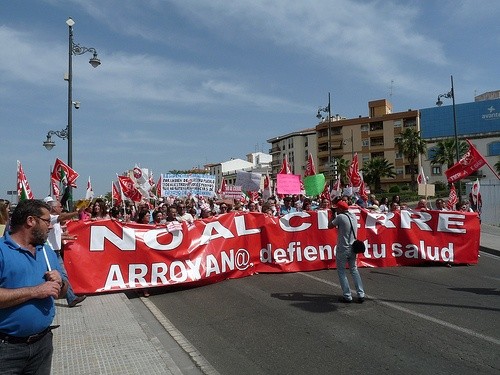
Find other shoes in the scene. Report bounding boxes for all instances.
[341,297,351,303]
[68,296,86,306]
[358,298,364,303]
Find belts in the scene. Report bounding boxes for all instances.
[0,328,51,344]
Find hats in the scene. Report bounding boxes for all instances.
[337,202,349,210]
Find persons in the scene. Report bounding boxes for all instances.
[0,199,67,375]
[0,187,482,308]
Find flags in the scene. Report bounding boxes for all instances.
[447,184,458,210]
[347,153,368,203]
[51,154,340,204]
[444,145,487,183]
[417,169,425,184]
[20,182,29,201]
[17,160,35,204]
[472,180,480,205]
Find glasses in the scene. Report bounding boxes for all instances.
[179,207,185,208]
[38,217,51,226]
[60,206,62,208]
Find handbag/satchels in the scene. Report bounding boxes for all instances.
[352,240,365,253]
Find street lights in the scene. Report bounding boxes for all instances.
[42,16,101,213]
[316,91,334,193]
[436,75,463,210]
